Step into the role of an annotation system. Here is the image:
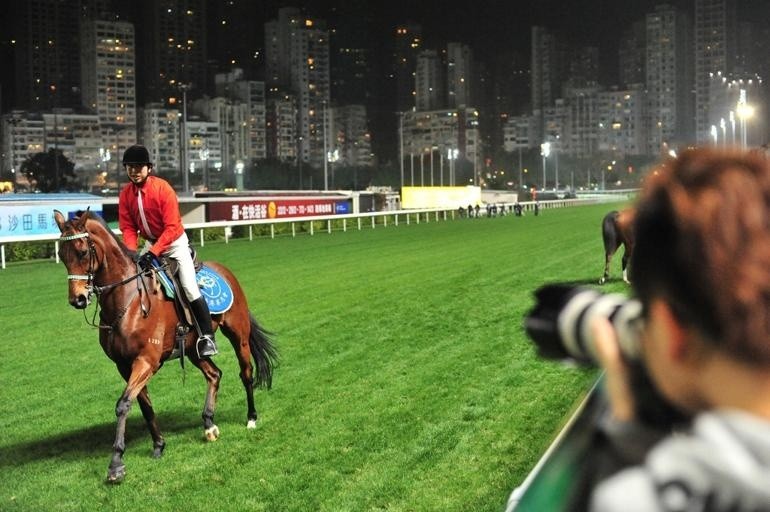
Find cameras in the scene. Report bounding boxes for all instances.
[525,282,697,428]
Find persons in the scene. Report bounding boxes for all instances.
[459,204,522,218]
[595,145,770,512]
[119,145,218,358]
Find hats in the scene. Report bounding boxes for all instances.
[122,145,152,166]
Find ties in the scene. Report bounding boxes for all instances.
[136,190,158,240]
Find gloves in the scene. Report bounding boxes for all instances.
[139,252,154,266]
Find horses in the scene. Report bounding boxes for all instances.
[53,205,283,486]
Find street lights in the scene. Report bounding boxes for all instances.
[709,87,754,151]
[328,149,339,189]
[540,142,552,191]
[448,147,460,187]
[98,146,112,188]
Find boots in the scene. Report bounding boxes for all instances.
[189,294,218,355]
